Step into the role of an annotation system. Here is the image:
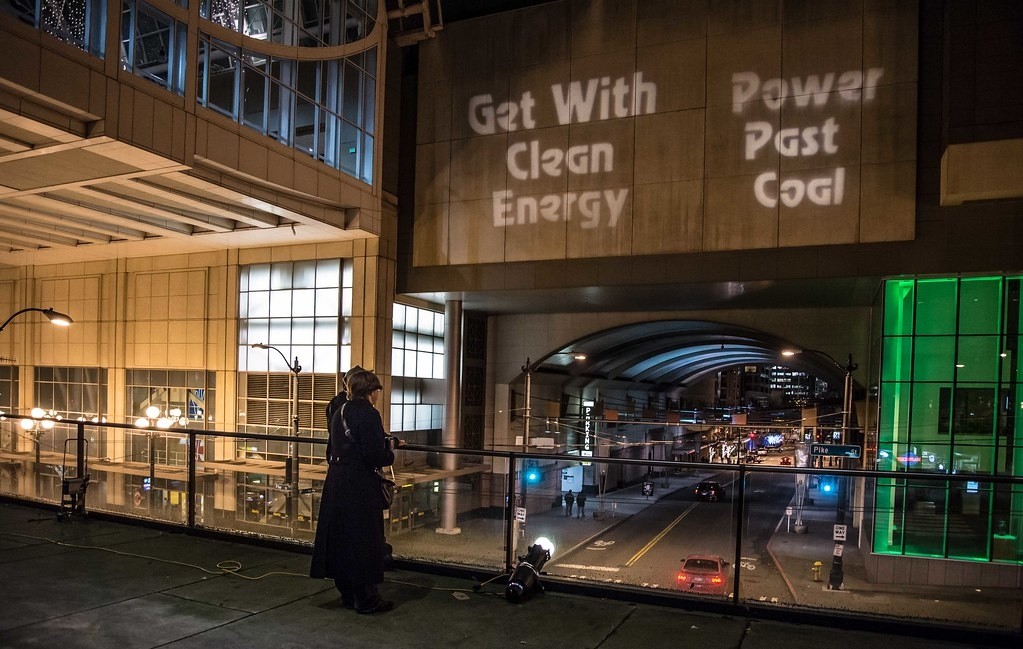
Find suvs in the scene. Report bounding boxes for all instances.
[695,480,727,503]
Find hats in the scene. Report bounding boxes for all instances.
[342,365,374,387]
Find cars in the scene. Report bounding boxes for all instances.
[743,447,768,464]
[780,456,792,465]
[676,553,731,596]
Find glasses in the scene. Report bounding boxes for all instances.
[377,384,383,392]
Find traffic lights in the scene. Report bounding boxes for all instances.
[528,462,537,480]
[822,476,833,494]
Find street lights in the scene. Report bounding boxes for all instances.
[135,404,172,517]
[165,407,190,519]
[782,346,860,593]
[20,407,54,499]
[519,350,590,538]
[251,342,303,538]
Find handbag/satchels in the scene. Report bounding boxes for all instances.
[380,477,396,509]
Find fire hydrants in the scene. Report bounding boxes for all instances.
[811,559,823,583]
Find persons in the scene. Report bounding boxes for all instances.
[701,456,709,463]
[565,490,574,516]
[309,366,407,612]
[576,490,586,518]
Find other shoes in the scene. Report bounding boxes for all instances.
[342,601,354,609]
[357,598,394,614]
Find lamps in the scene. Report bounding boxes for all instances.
[504,544,551,604]
